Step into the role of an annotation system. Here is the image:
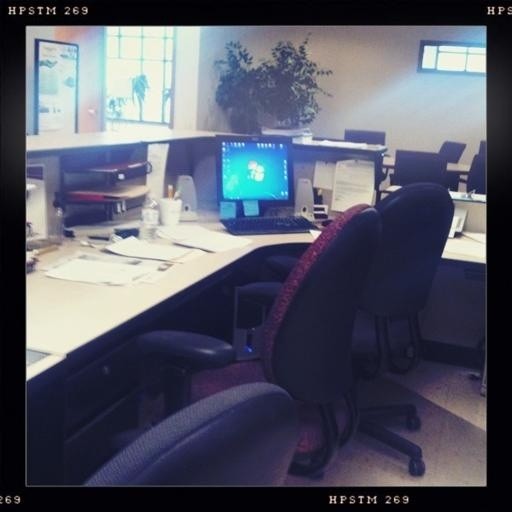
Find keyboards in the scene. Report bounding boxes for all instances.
[220,216,318,235]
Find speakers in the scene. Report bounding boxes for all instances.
[176,175,198,221]
[294,178,315,222]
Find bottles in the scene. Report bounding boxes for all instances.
[48,207,64,243]
[141,192,160,227]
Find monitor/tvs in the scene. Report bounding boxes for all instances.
[216,135,293,217]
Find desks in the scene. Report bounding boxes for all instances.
[27,196,489,476]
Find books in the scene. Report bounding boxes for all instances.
[72,184,147,198]
[71,161,147,172]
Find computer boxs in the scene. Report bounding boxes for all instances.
[213,279,267,362]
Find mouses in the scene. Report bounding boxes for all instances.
[322,220,332,226]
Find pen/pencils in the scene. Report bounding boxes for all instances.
[168,185,180,201]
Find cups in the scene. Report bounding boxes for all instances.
[161,197,182,226]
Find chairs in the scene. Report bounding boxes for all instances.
[82,128,487,489]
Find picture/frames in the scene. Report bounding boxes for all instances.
[32,38,81,138]
